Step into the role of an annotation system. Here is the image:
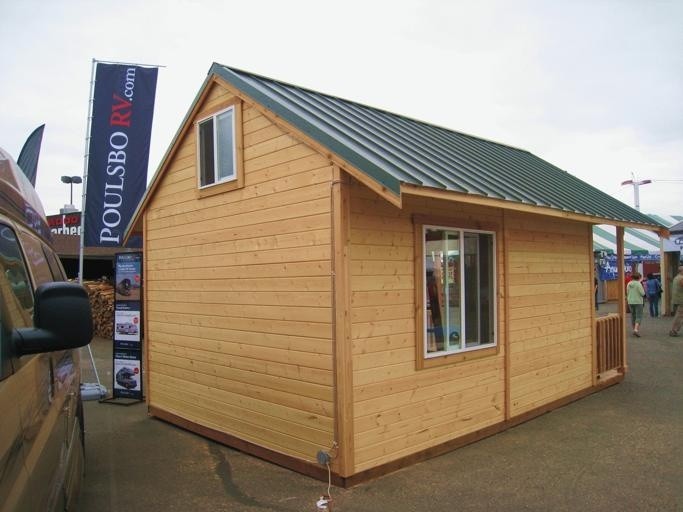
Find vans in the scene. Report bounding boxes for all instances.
[0,149,89,511]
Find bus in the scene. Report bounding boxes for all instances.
[118,279,131,296]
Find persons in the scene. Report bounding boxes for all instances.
[644,272,661,317]
[626,272,645,337]
[669,266,683,336]
[625,271,633,313]
[594,275,599,311]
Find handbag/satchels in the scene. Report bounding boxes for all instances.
[655,286,662,298]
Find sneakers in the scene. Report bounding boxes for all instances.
[633,329,641,337]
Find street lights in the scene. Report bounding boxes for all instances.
[619,178,651,211]
[60,175,83,206]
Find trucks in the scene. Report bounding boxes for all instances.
[116,368,137,389]
[116,323,138,334]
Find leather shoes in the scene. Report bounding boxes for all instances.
[668,330,679,336]
